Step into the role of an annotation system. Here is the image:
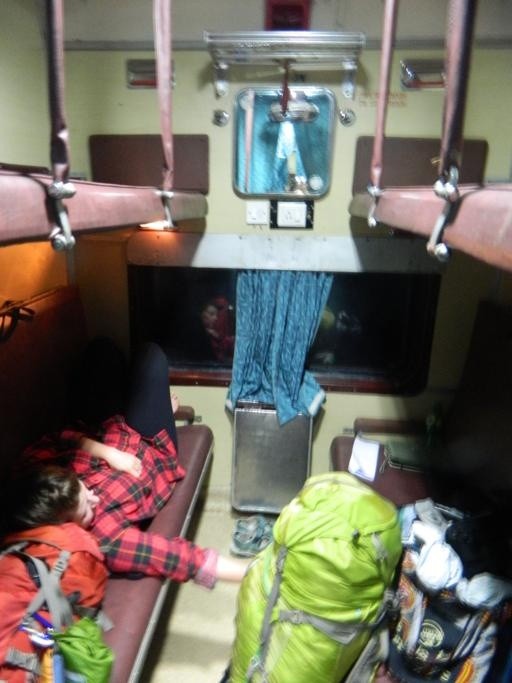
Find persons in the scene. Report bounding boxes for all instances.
[199,298,235,365]
[0,342,249,592]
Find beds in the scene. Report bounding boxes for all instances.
[346,184,508,284]
[2,159,212,258]
[331,416,508,511]
[2,420,212,681]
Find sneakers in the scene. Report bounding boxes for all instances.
[228,515,277,558]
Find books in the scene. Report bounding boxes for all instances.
[382,440,427,475]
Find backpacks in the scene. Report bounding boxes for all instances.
[348,499,510,683]
[0,523,113,682]
[224,471,404,683]
